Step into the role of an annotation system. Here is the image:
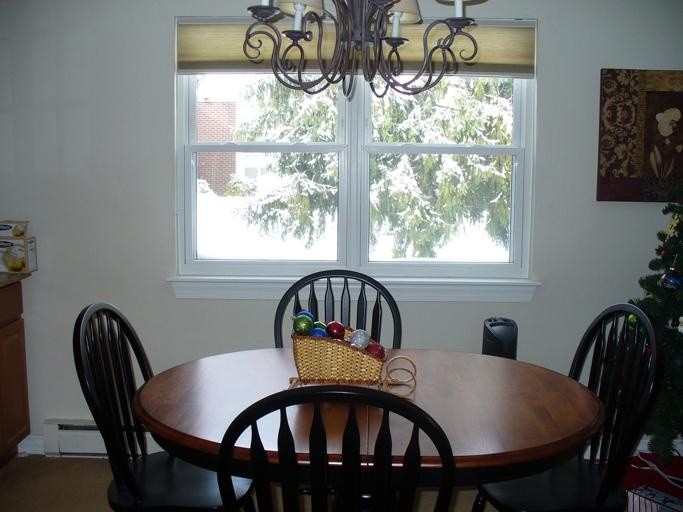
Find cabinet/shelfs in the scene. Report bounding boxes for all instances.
[0,271,31,468]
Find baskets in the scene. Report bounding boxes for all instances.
[290,322,388,385]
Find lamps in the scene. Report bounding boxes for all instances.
[243,0,486,96]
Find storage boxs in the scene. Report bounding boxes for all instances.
[0,237,37,274]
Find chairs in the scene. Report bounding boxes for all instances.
[218,385,457,512]
[473,304,661,512]
[275,269,402,350]
[73,303,257,512]
